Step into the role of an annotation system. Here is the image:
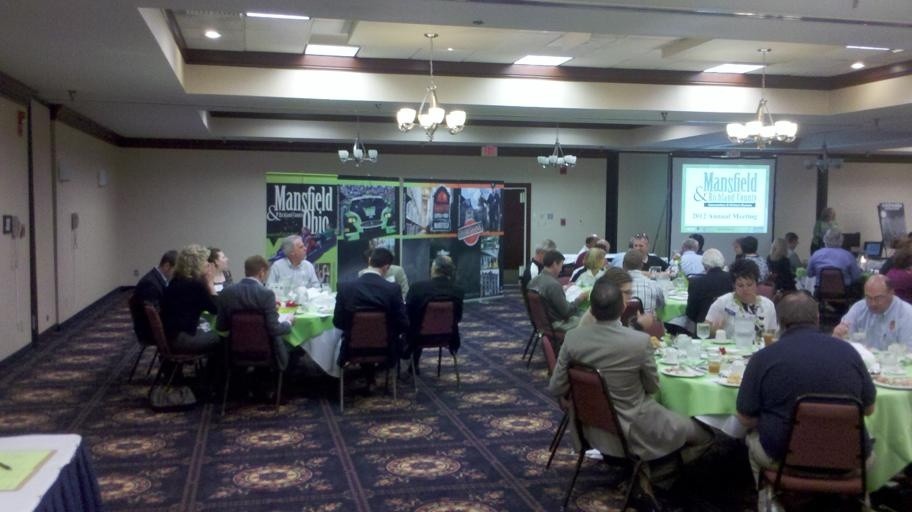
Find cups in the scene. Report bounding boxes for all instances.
[853,332,866,345]
[649,266,687,300]
[655,321,776,378]
[878,350,897,371]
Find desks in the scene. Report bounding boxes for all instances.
[0,431,104,512]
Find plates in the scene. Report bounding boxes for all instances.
[872,377,912,390]
[717,377,740,387]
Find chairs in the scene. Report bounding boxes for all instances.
[520,263,869,467]
[220,312,284,419]
[339,304,400,415]
[411,298,463,390]
[129,277,418,393]
[558,360,654,510]
[145,301,210,415]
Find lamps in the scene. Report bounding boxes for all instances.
[336,115,379,168]
[536,123,577,169]
[802,131,844,173]
[723,48,799,146]
[395,31,467,138]
